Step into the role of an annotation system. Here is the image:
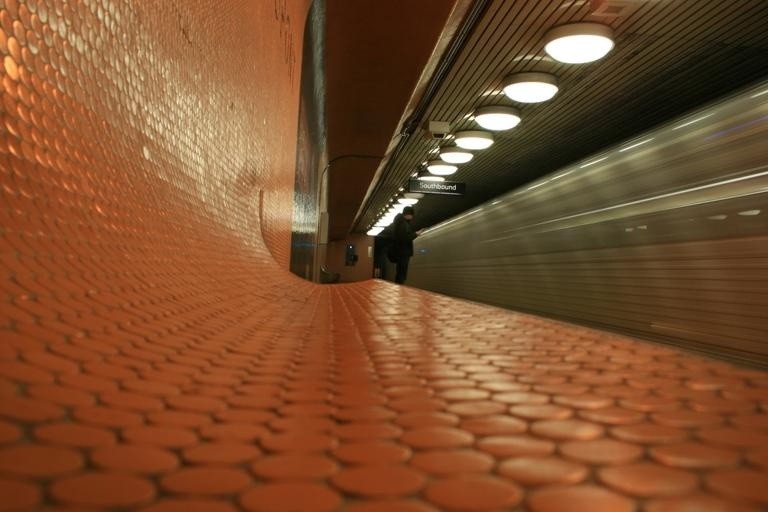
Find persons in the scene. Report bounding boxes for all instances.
[389,205,425,284]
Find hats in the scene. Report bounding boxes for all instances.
[404,206,414,216]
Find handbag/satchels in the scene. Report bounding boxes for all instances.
[387,241,399,263]
